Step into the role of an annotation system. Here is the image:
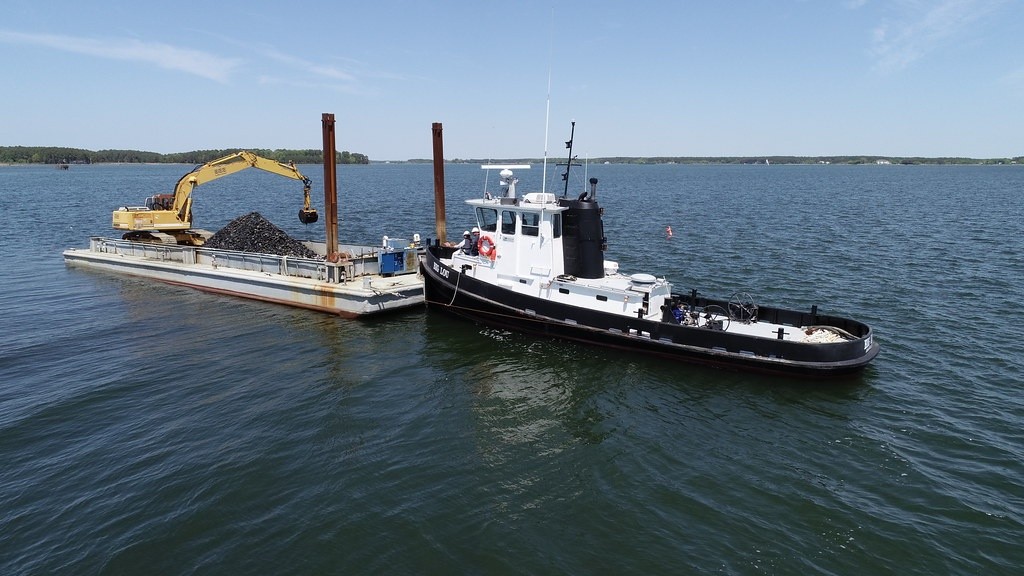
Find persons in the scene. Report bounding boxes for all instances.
[462,231,472,255]
[469,227,479,256]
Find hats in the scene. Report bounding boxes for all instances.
[463,231,470,236]
[471,227,479,232]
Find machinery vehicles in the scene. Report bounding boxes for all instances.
[111,150,318,253]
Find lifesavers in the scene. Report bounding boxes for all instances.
[477,236,495,255]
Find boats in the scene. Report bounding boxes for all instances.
[418,71,883,380]
